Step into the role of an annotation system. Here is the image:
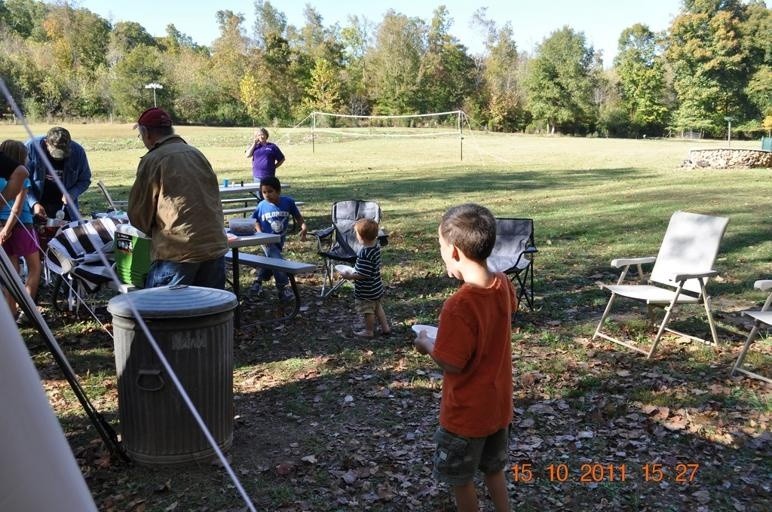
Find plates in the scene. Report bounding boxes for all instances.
[411,323,438,344]
[335,263,359,274]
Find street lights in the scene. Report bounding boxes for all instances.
[145,82,165,110]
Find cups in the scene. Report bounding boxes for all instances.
[223,178,230,188]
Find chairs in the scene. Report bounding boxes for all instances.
[590,210,731,363]
[483,217,540,317]
[728,279,772,388]
[315,196,389,297]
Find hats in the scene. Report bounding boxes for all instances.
[44,126,71,160]
[132,107,172,129]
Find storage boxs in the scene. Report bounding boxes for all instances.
[113,232,154,288]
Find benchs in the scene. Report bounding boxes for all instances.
[61,268,139,300]
[219,197,257,205]
[221,200,306,227]
[224,249,317,324]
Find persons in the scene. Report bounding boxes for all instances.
[414,203,518,512]
[128,107,229,291]
[0,146,30,212]
[0,140,47,326]
[245,128,285,202]
[1,188,27,244]
[337,218,391,338]
[249,176,307,301]
[23,127,93,225]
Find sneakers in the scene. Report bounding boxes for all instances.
[278,288,296,303]
[249,282,262,297]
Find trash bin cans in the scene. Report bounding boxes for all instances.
[106,284,238,464]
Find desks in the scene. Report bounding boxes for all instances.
[216,182,290,200]
[216,222,282,328]
